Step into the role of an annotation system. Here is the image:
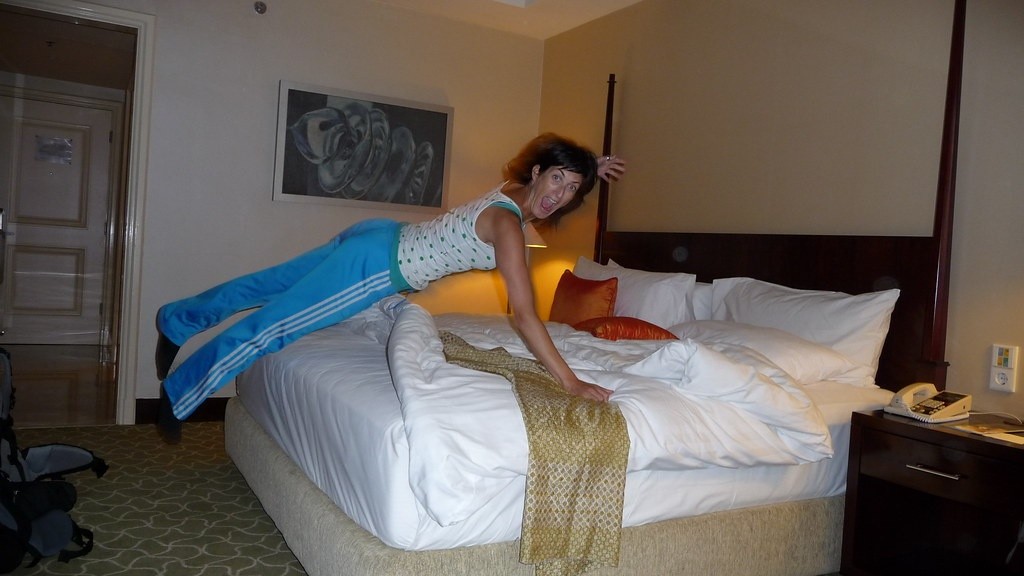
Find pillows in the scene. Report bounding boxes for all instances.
[712,277,900,382]
[573,315,679,340]
[605,259,714,323]
[667,320,857,384]
[570,255,697,331]
[548,269,619,328]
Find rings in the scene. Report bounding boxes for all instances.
[607,155,610,161]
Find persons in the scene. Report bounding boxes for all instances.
[155,134,625,447]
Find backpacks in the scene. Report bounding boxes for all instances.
[0,346,110,576]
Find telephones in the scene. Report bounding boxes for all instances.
[883,383,972,424]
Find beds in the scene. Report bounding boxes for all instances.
[222,0,967,576]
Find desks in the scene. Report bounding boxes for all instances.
[838,409,1024,576]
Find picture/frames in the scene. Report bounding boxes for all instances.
[272,79,455,217]
